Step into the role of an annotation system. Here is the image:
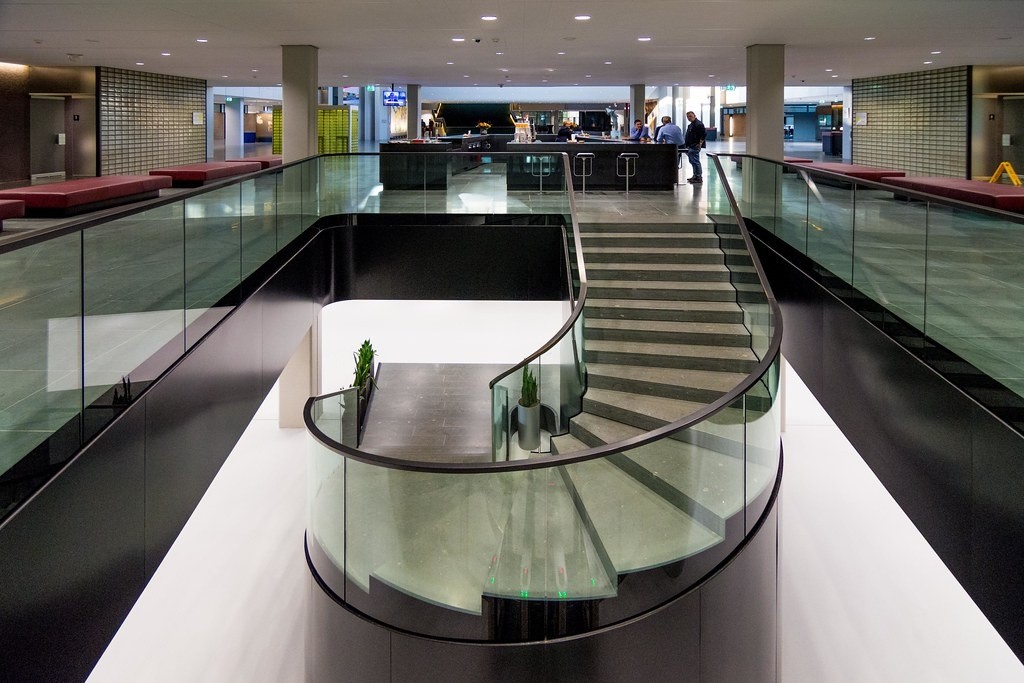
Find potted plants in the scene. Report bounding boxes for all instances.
[517,363,541,450]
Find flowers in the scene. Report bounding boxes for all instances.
[475,122,492,130]
[562,120,578,132]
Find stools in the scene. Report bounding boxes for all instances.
[616,152,639,197]
[573,152,595,197]
[532,154,551,196]
[678,148,688,170]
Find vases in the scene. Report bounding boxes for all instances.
[480,129,487,135]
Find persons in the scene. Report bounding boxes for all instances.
[555,120,581,142]
[386,93,399,105]
[421,119,434,137]
[630,111,707,183]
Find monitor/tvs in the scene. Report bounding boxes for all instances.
[383,91,406,107]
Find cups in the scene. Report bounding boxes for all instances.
[618,131,621,138]
[611,131,614,139]
[602,132,605,136]
[571,134,575,140]
[515,133,524,142]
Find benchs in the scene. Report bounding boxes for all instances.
[227,155,282,168]
[0,175,175,219]
[731,156,812,174]
[149,162,261,186]
[880,176,1024,212]
[792,162,904,189]
[0,200,25,232]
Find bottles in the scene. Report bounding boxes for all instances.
[426,131,429,140]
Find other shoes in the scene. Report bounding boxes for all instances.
[687,176,702,183]
